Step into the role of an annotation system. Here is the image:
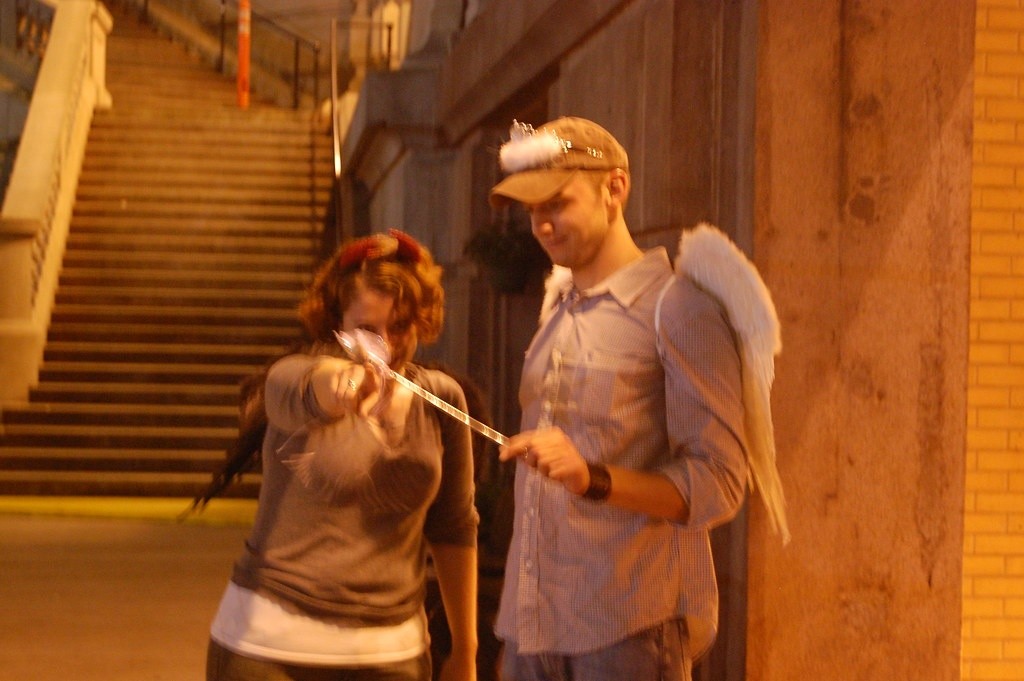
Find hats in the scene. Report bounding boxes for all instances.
[489,116,629,203]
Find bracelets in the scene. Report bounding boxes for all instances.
[583,462,611,500]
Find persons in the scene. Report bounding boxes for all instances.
[492,115,747,681]
[206,232,481,681]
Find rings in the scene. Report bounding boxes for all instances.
[348,379,356,390]
